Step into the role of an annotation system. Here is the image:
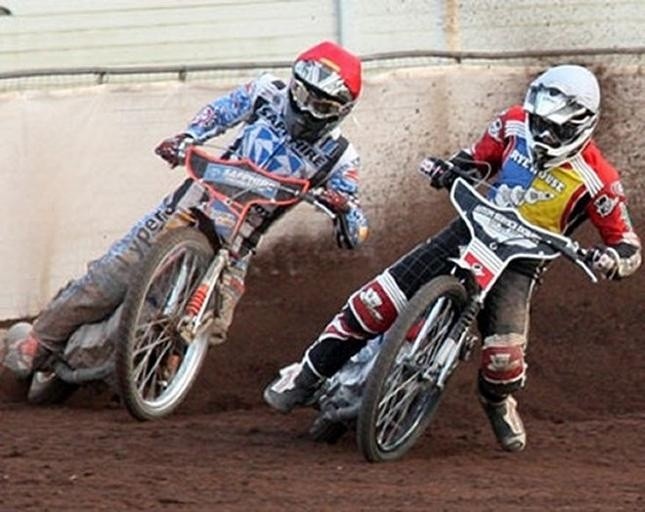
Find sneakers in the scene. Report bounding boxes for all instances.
[2,335,39,380]
[263,362,316,416]
[477,393,527,454]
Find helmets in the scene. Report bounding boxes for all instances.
[285,42,361,143]
[521,64,601,171]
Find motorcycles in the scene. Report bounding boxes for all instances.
[4,130,358,424]
[309,147,616,462]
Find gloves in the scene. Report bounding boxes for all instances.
[158,133,189,170]
[419,157,456,188]
[337,208,369,249]
[576,247,616,283]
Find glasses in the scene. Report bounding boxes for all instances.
[530,112,578,140]
[288,78,343,119]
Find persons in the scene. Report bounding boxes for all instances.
[1,33,371,409]
[257,62,645,458]
